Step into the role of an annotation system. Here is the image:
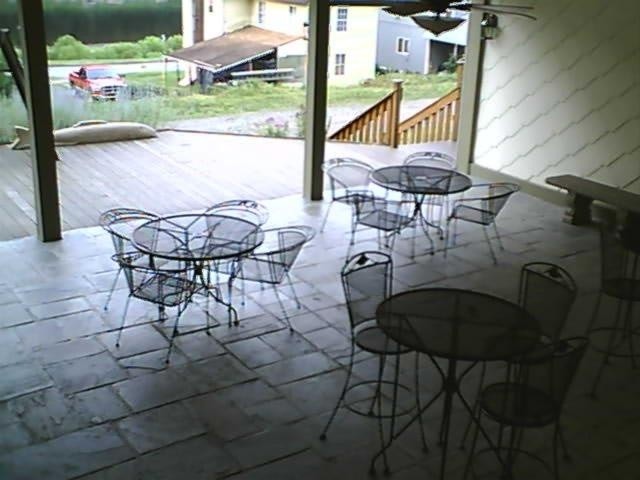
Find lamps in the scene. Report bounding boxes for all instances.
[411,12,465,36]
[481,13,498,39]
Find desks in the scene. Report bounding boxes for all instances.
[373,288,541,449]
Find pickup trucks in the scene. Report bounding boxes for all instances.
[68,64,130,102]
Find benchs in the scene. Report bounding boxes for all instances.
[545,175,639,228]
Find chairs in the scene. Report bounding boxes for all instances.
[321,151,522,262]
[574,221,640,400]
[461,261,576,465]
[100,200,317,364]
[463,335,591,479]
[319,251,431,454]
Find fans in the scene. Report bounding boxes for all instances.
[329,1,538,23]
